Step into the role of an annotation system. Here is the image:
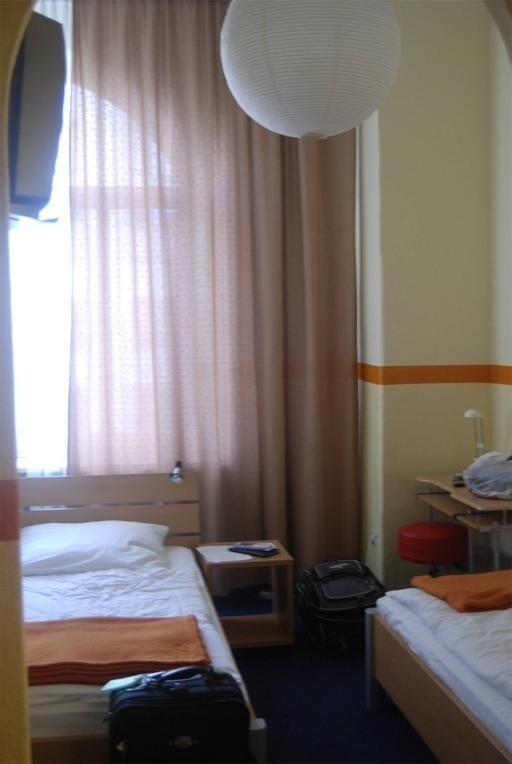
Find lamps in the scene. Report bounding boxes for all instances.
[462,408,487,458]
[170,461,187,487]
[218,0,410,141]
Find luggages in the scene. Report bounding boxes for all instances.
[294,558,385,654]
[103,663,251,764]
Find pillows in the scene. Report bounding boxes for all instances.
[17,521,171,574]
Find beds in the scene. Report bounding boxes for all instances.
[18,461,268,763]
[363,569,511,763]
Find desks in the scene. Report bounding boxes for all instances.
[195,539,294,649]
[416,471,512,575]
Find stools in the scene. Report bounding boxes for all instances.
[396,521,468,576]
